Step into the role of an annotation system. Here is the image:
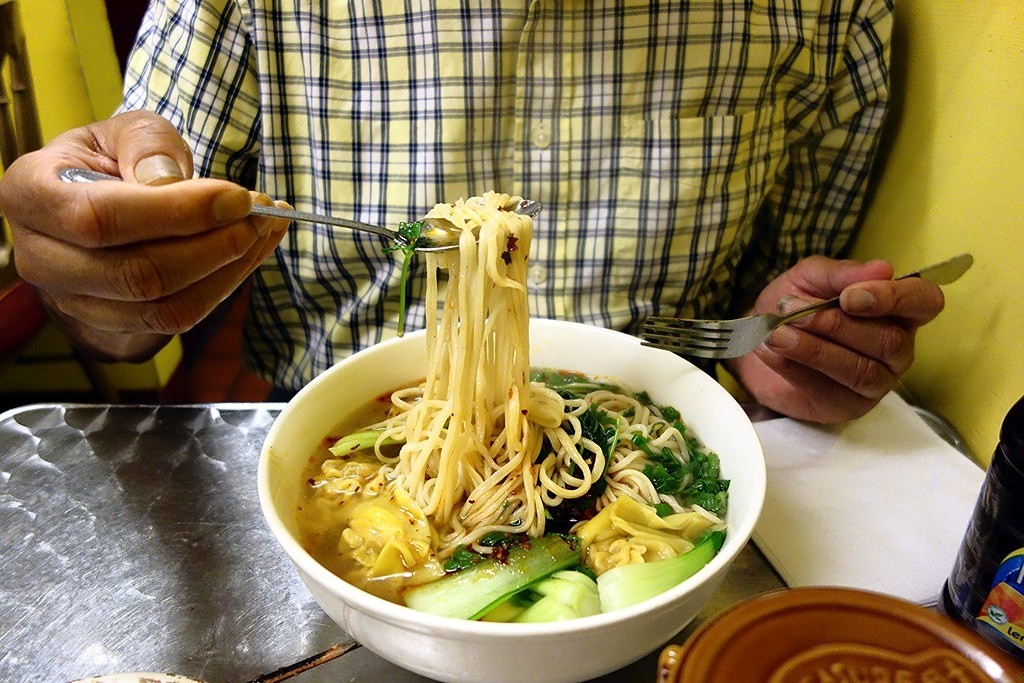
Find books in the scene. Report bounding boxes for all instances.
[751,389,988,618]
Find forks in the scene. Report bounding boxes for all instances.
[639,254,975,358]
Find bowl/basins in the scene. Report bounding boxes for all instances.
[658,587,1023,683]
[258,318,766,683]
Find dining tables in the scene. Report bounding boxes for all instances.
[0,402,1024,683]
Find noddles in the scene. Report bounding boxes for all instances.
[298,188,732,624]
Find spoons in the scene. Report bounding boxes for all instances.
[60,167,543,252]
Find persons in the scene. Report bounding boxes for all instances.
[2,0,951,433]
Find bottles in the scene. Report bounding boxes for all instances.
[937,397,1024,668]
